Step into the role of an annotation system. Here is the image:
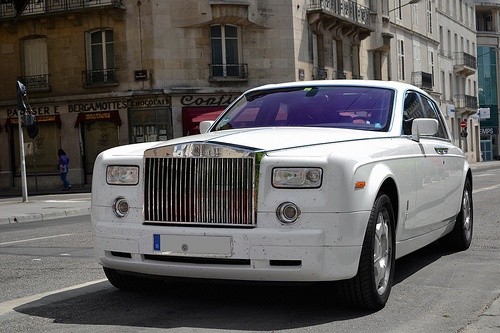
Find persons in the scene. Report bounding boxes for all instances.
[57,149,72,192]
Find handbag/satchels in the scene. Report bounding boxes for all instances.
[60,164,67,173]
[57,161,61,170]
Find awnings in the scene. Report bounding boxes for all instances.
[181,107,289,130]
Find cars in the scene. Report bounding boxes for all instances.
[90,79,474,314]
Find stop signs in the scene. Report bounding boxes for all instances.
[460,120,467,128]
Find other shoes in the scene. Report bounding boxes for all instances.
[68,184,72,188]
[61,188,69,191]
